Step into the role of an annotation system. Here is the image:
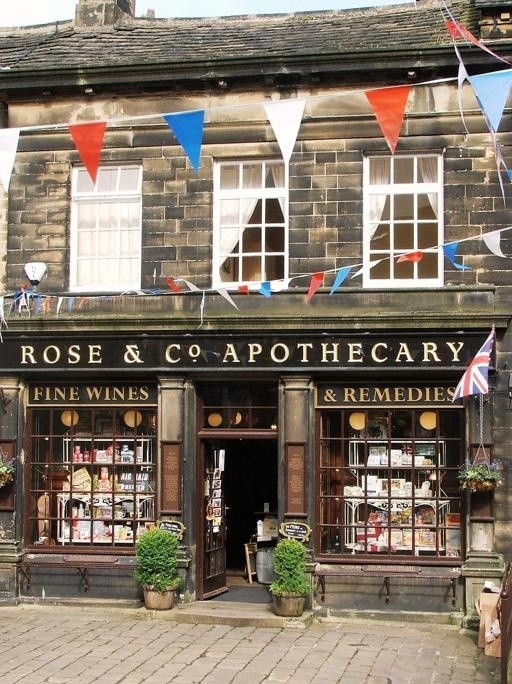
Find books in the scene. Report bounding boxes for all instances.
[57,442,150,543]
[354,446,436,547]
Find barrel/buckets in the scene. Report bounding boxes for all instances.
[272,589,306,617]
[142,582,178,611]
[255,546,276,585]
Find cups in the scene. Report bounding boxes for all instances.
[110,449,120,455]
[114,505,126,519]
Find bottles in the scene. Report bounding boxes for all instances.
[77,502,91,519]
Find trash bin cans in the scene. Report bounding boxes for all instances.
[255,541,277,585]
[460,551,507,628]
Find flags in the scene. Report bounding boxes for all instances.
[451,329,495,404]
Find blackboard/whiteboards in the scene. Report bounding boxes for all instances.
[156,519,187,541]
[279,521,312,543]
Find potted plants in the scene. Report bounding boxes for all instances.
[267,536,314,616]
[132,526,186,610]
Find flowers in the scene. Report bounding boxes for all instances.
[457,455,506,494]
[0,446,16,488]
